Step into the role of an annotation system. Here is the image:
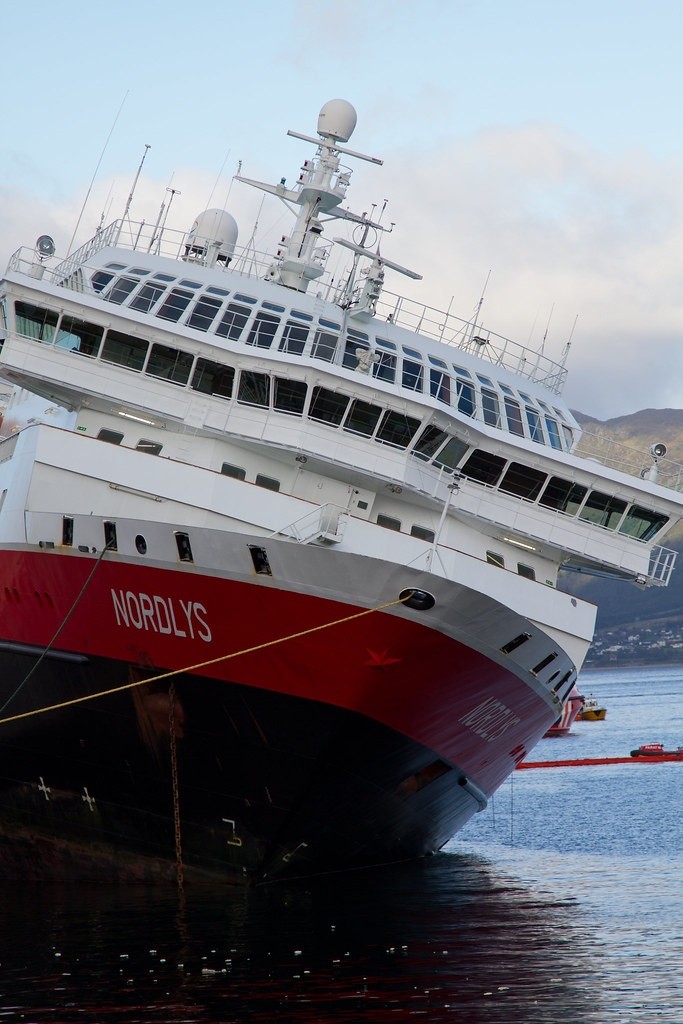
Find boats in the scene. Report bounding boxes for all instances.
[5,100,683,927]
[574,694,607,720]
[546,684,585,731]
[630,744,663,756]
